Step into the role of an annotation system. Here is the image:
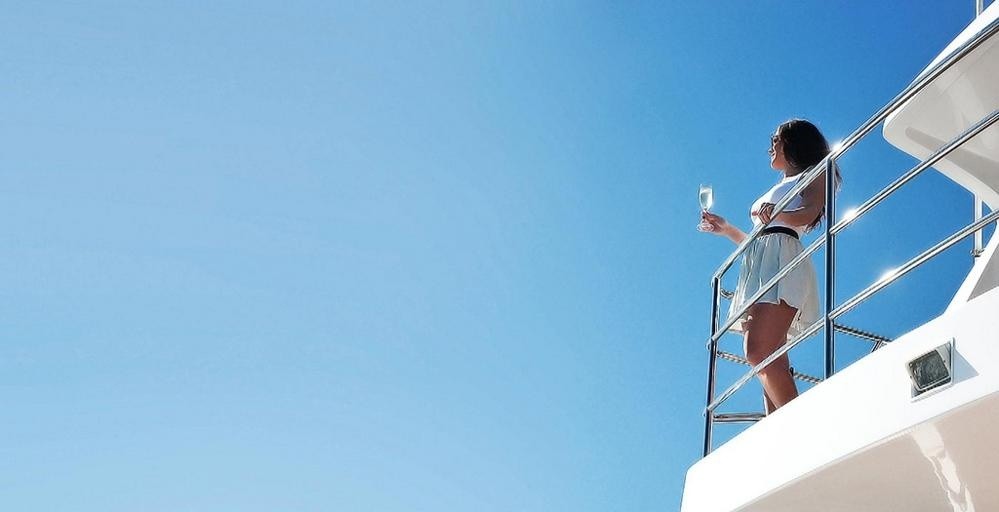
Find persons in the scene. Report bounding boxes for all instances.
[702,120,842,417]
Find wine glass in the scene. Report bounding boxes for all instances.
[695,181,715,234]
